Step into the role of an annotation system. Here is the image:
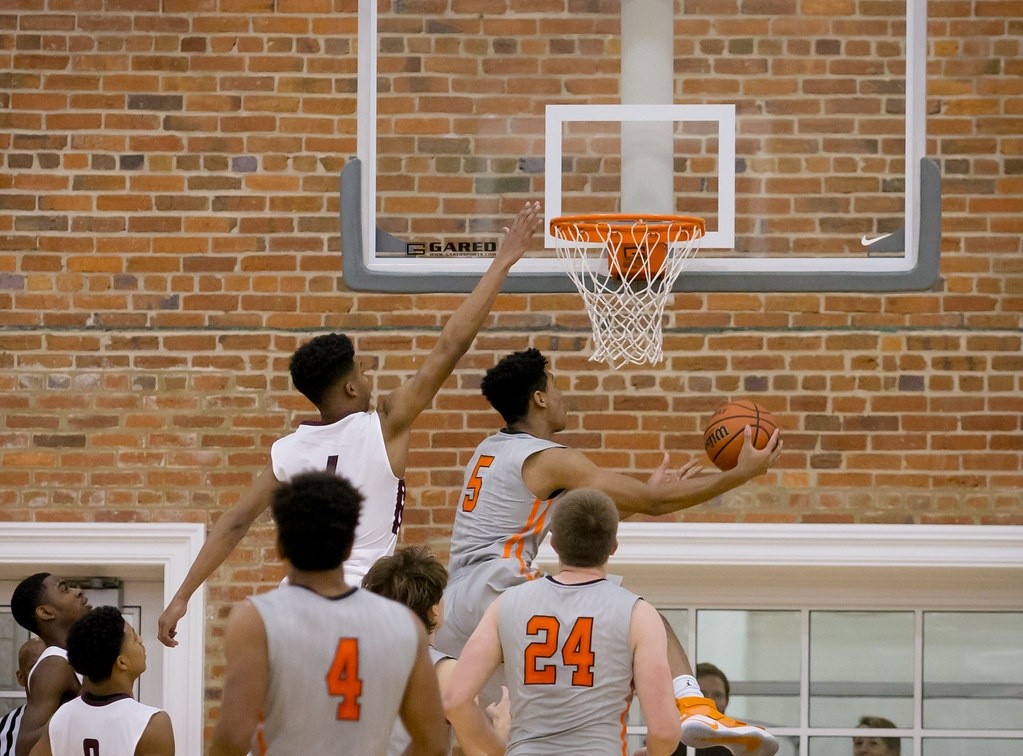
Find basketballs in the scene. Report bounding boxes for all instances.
[702,400,779,472]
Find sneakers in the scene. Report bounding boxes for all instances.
[675,696,779,756]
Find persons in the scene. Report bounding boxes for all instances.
[671,663,733,756]
[0,572,93,756]
[360,547,511,756]
[434,348,782,756]
[158,200,543,756]
[853,717,900,756]
[28,605,174,756]
[207,471,450,756]
[442,488,681,756]
